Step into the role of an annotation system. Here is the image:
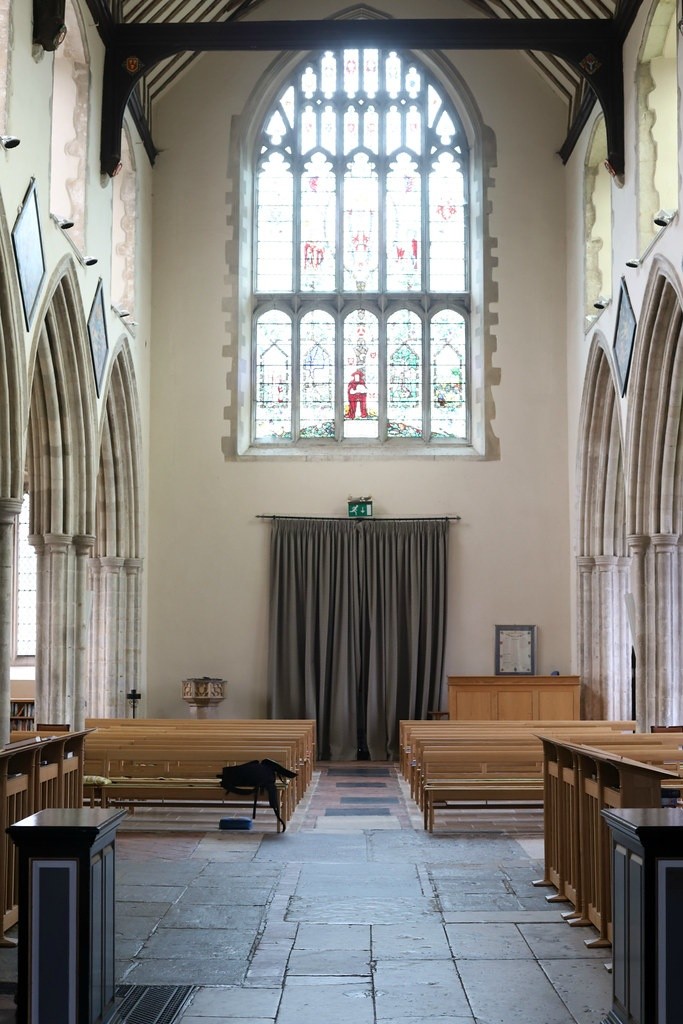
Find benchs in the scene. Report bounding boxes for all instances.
[399,719,683,833]
[84,718,314,830]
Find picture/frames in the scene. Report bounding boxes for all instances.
[497,624,536,674]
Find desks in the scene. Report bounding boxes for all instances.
[600,808,683,1024]
[7,808,128,1024]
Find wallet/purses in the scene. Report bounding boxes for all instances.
[219,817,252,830]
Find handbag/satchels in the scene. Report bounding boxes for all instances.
[216,759,298,832]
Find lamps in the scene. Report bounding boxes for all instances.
[59,218,73,230]
[0,134,21,148]
[626,258,640,268]
[653,210,672,227]
[84,255,97,264]
[594,297,609,310]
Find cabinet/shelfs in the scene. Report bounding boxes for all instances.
[11,698,35,731]
[450,677,582,720]
